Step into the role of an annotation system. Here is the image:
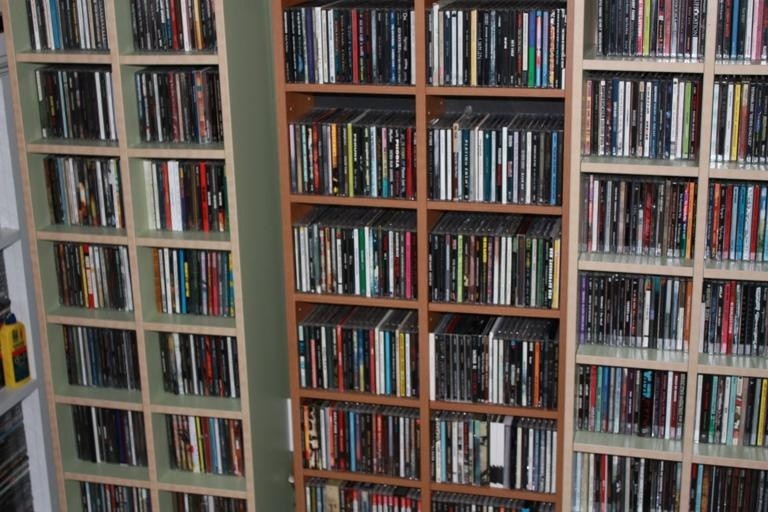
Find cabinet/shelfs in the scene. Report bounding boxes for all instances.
[271,0,573,512]
[0,0,294,512]
[562,0,768,512]
[0,32,59,512]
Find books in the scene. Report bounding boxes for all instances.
[281,1,768,512]
[0,0,248,511]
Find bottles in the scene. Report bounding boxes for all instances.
[2,314,32,387]
